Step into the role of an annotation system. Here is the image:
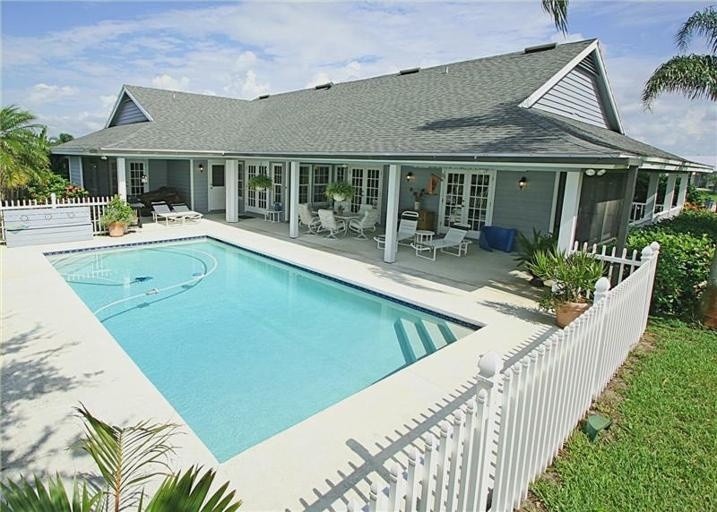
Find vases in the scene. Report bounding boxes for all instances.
[414,202,421,210]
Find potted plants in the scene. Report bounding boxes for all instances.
[511,228,611,328]
[248,174,273,191]
[99,193,137,237]
[325,182,357,202]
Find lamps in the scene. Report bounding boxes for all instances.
[518,176,526,192]
[405,172,412,183]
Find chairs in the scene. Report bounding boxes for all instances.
[297,202,378,242]
[414,222,473,260]
[150,199,204,227]
[373,210,419,250]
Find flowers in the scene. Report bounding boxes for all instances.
[409,186,427,201]
